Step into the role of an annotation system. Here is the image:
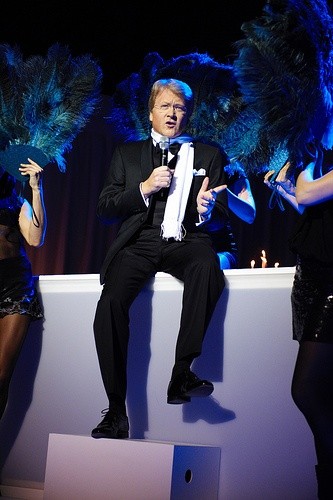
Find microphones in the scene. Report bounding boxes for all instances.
[158,136,169,197]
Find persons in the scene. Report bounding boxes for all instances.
[91,79,228,439]
[291,162,333,500]
[217,167,256,270]
[0,158,47,419]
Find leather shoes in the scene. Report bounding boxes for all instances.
[167,369,214,405]
[92,406,130,438]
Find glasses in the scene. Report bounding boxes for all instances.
[154,104,188,113]
